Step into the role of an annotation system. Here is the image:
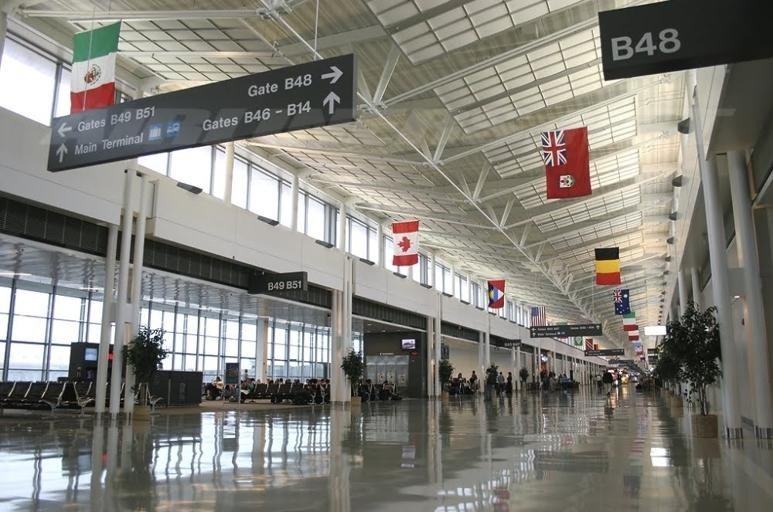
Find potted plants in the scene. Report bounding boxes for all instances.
[651,300,723,438]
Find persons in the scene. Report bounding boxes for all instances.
[452,365,621,400]
[203,370,399,408]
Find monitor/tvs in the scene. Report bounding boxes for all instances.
[84,347,98,362]
[400,337,417,350]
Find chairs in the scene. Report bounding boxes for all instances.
[0,377,163,420]
[241,384,399,404]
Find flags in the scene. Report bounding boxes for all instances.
[612,288,630,317]
[585,338,593,351]
[67,16,119,114]
[593,344,598,351]
[592,245,621,288]
[538,126,593,204]
[572,335,583,347]
[388,218,421,269]
[622,309,647,363]
[555,320,569,339]
[484,276,507,310]
[530,306,548,329]
